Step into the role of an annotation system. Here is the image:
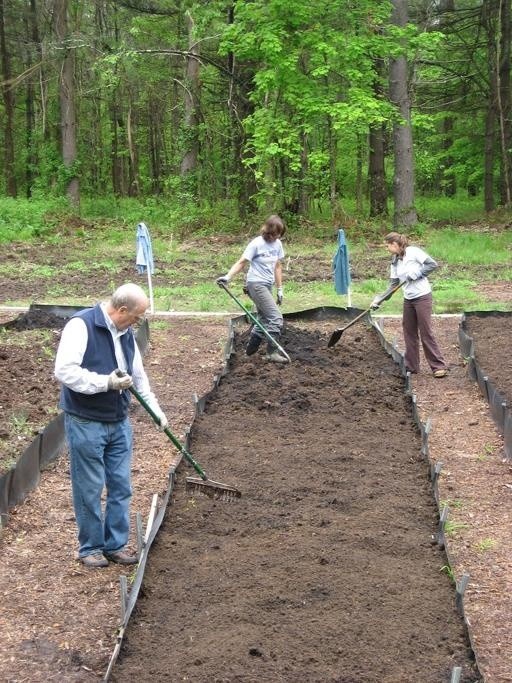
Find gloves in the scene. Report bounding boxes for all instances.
[108,368,133,390]
[370,296,383,311]
[216,275,229,288]
[276,288,283,305]
[405,269,422,283]
[149,411,169,432]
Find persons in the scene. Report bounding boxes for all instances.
[212,213,288,365]
[368,230,449,380]
[52,281,172,568]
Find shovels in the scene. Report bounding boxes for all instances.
[328,281,406,347]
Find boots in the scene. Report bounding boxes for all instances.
[266,332,288,364]
[244,332,265,360]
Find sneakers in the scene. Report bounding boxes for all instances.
[81,553,108,566]
[107,551,138,565]
[434,369,450,377]
